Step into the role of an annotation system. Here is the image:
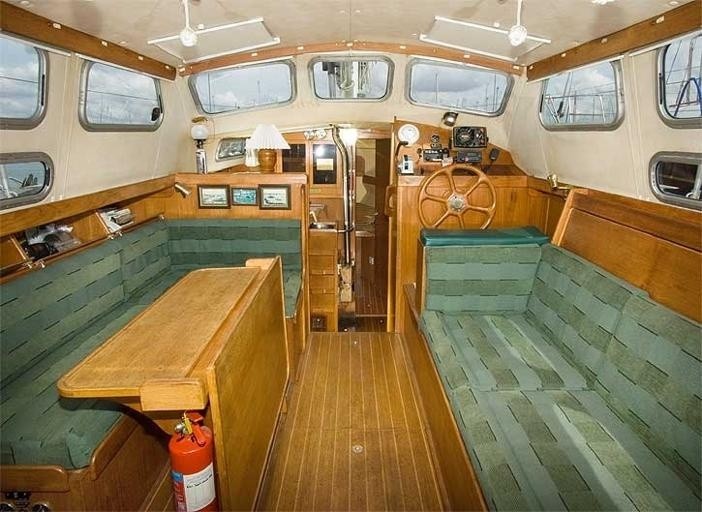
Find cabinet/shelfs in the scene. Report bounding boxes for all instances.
[309,233,338,332]
[277,134,344,200]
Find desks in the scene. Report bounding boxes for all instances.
[56,255,290,511]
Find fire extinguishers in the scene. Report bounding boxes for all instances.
[168,411,218,512]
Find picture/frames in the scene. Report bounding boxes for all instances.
[258,184,291,210]
[231,187,258,206]
[197,184,230,209]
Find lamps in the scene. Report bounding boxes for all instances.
[190,124,209,174]
[509,0,528,46]
[243,123,292,175]
[180,0,197,47]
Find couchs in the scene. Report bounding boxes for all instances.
[415,224,702,512]
[0,215,312,511]
